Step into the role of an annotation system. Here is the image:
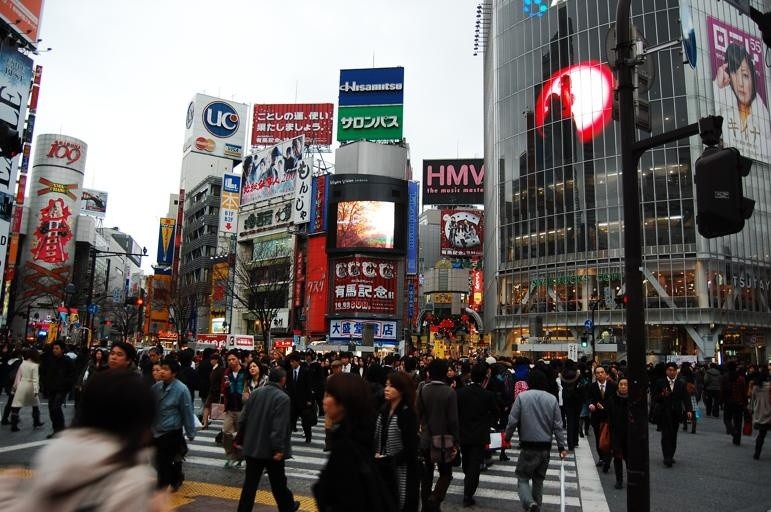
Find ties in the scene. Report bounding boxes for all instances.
[600,384,605,399]
[293,371,296,383]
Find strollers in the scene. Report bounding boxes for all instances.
[216,427,244,468]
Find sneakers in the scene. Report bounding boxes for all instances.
[526,503,538,512]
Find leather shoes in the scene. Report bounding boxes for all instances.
[463,496,475,507]
[603,466,610,472]
[615,480,623,490]
[291,501,300,512]
[595,461,603,467]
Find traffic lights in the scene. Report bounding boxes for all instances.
[580,337,586,349]
[621,295,628,307]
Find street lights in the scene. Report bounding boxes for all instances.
[737,152,755,225]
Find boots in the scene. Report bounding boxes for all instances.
[33,414,43,429]
[10,415,19,432]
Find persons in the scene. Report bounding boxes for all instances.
[0,367,173,512]
[1,337,768,489]
[457,361,505,511]
[244,139,305,184]
[499,295,577,315]
[417,359,461,511]
[312,374,401,512]
[39,201,73,238]
[370,372,419,512]
[224,367,302,511]
[445,213,477,246]
[503,361,568,512]
[712,40,770,155]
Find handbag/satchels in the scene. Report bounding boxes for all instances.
[210,396,225,420]
[429,434,456,465]
[695,401,701,418]
[742,408,752,436]
[598,421,613,453]
